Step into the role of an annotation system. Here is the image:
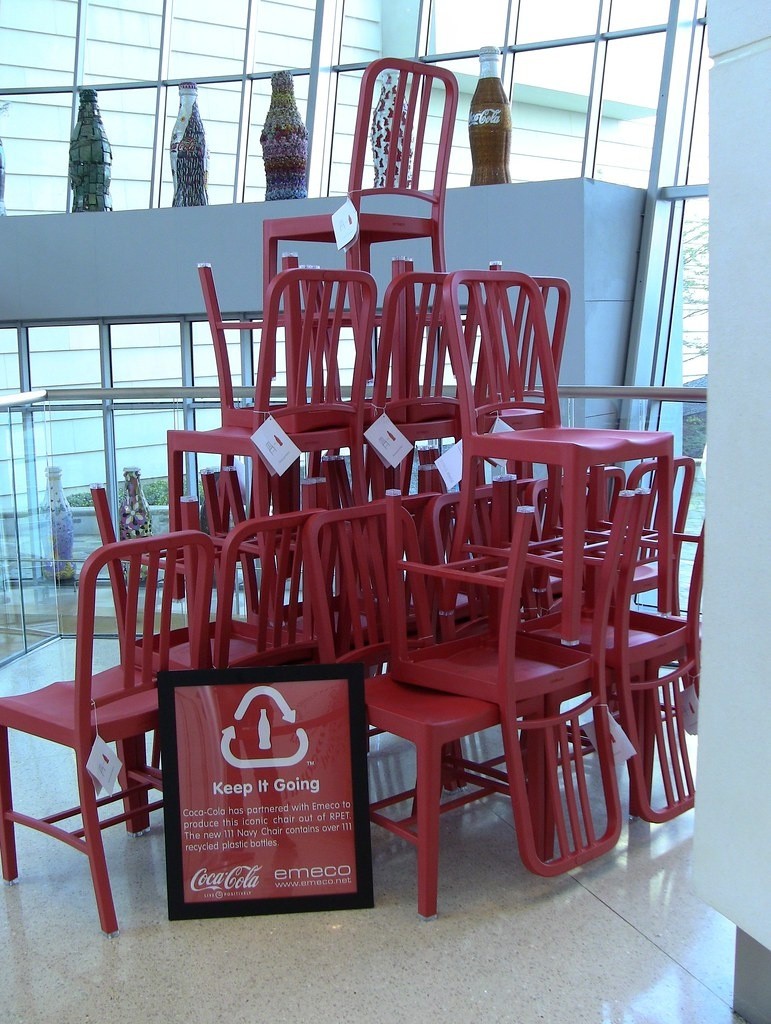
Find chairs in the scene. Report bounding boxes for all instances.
[0,57,707,927]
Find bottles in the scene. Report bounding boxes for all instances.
[170,81,209,207]
[260,71,309,201]
[370,68,411,189]
[40,466,76,581]
[0,137,7,217]
[201,472,220,534]
[468,46,512,186]
[118,466,151,585]
[69,90,112,212]
